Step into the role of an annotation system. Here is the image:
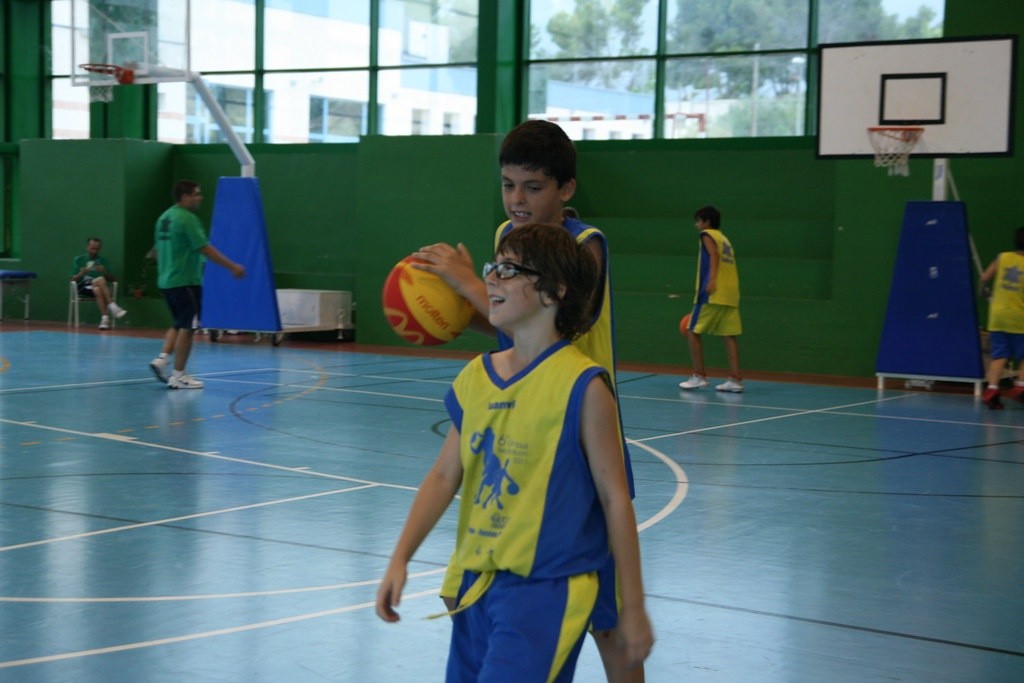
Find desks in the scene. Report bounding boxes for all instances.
[0,270,36,322]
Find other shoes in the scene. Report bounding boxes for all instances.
[113,306,128,319]
[98,321,110,329]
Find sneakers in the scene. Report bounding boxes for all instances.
[983,388,1004,411]
[167,374,203,389]
[1008,386,1024,401]
[150,357,170,382]
[715,380,744,392]
[679,375,708,389]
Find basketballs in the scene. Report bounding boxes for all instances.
[383,256,471,349]
[679,313,692,335]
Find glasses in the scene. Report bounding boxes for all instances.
[483,262,541,279]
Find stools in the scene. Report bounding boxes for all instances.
[68,280,118,330]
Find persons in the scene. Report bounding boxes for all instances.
[149,179,246,390]
[980,226,1024,407]
[374,222,656,682]
[69,237,127,329]
[411,119,646,683]
[679,206,745,393]
